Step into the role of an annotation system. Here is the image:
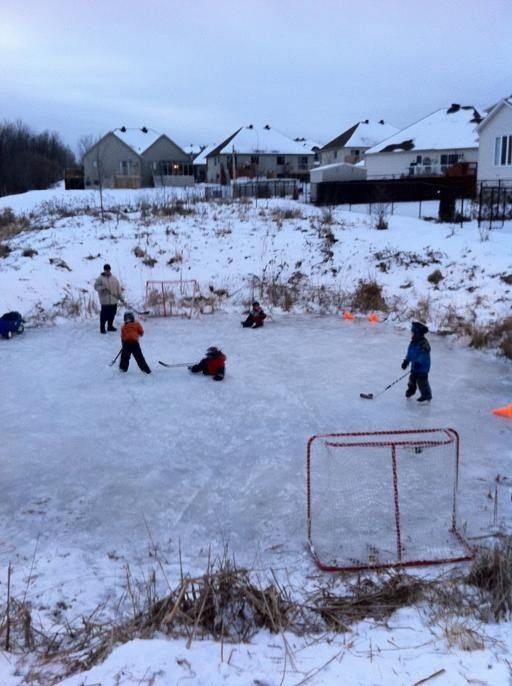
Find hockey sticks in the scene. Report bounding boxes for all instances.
[359,370,411,400]
[158,361,198,367]
[106,289,150,315]
[109,349,122,366]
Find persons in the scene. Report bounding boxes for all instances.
[191,347,226,380]
[94,263,127,334]
[240,301,267,329]
[401,321,432,403]
[118,311,152,374]
